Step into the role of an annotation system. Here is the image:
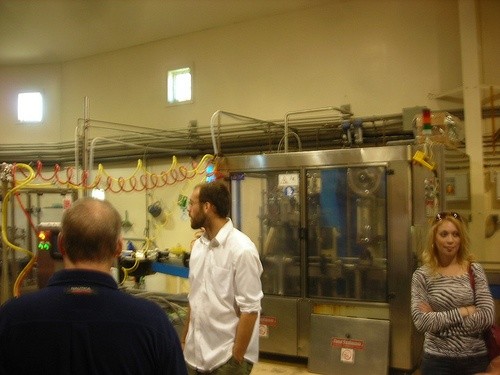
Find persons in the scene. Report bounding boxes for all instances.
[410,211,495,375]
[0,197,188,375]
[183,182,264,375]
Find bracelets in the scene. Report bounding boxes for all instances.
[465,306,470,316]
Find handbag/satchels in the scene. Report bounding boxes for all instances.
[482,323,500,360]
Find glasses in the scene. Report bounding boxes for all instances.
[432,212,462,223]
